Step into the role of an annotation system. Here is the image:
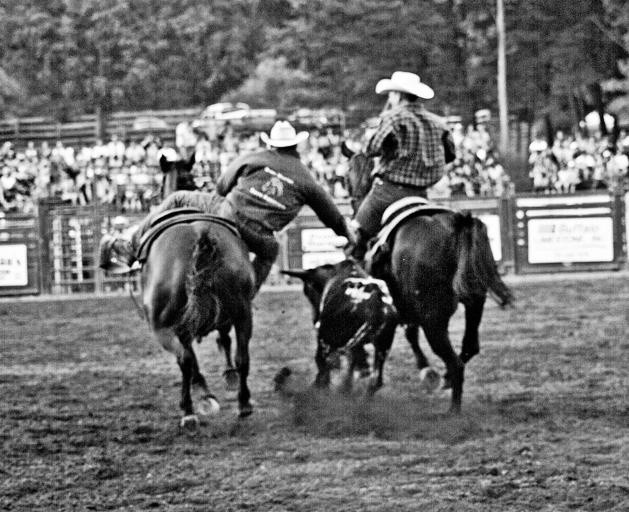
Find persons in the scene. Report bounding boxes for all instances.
[1,121,267,214]
[529,130,628,193]
[425,125,512,199]
[300,124,373,199]
[350,71,458,271]
[111,120,355,301]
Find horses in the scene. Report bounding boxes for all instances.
[338,139,519,416]
[136,150,256,438]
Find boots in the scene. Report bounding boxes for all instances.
[344,229,372,259]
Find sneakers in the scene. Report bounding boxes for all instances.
[112,240,135,265]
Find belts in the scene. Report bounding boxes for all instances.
[236,211,273,235]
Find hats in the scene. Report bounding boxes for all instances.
[375,71,435,100]
[260,120,309,147]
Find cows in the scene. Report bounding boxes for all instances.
[279,259,401,404]
